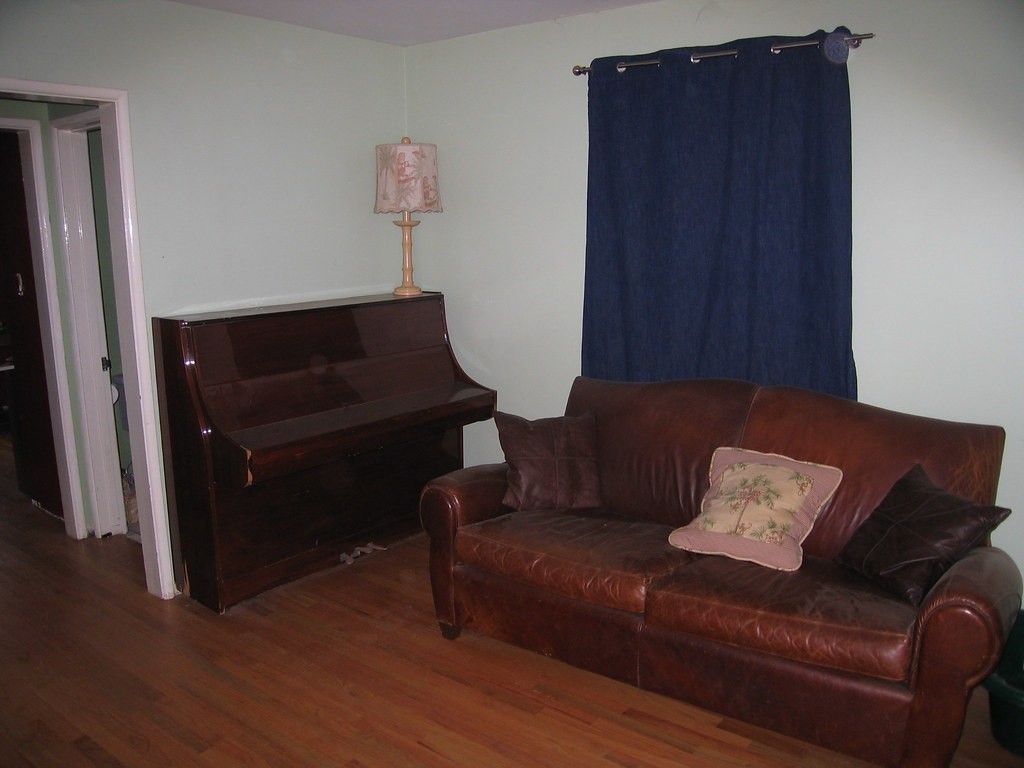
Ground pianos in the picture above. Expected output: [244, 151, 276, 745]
[150, 289, 498, 616]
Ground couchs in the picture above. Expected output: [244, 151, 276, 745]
[418, 376, 1024, 768]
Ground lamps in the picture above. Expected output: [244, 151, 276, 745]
[374, 135, 445, 297]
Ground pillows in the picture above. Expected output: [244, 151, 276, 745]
[492, 411, 603, 512]
[666, 447, 843, 572]
[831, 462, 1012, 608]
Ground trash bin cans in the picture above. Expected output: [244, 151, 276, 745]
[985, 607, 1024, 754]
